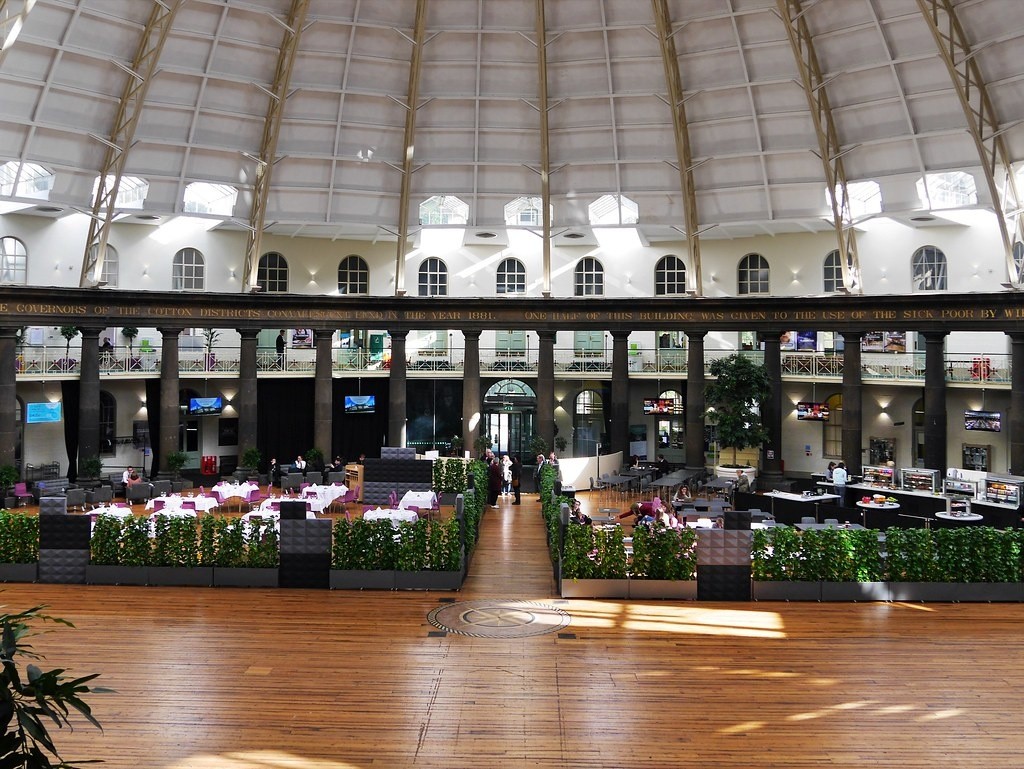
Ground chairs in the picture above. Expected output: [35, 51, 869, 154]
[14, 465, 443, 539]
[585, 465, 885, 539]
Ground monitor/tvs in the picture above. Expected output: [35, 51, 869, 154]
[189, 397, 223, 416]
[26, 402, 62, 424]
[344, 395, 377, 414]
[965, 410, 1001, 432]
[643, 398, 674, 416]
[797, 402, 830, 421]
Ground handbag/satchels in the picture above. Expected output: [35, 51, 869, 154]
[512, 480, 518, 487]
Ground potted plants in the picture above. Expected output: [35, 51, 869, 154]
[166, 449, 190, 493]
[239, 447, 261, 481]
[699, 353, 773, 484]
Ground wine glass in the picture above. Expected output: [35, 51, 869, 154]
[161, 492, 166, 498]
[99, 501, 105, 509]
[187, 492, 194, 499]
[62, 487, 65, 495]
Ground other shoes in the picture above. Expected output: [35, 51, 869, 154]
[490, 505, 500, 509]
[536, 499, 540, 502]
[512, 502, 520, 505]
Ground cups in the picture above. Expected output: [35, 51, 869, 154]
[802, 493, 808, 499]
[253, 505, 259, 512]
[223, 481, 227, 487]
[235, 480, 239, 486]
[270, 492, 276, 500]
[298, 493, 303, 499]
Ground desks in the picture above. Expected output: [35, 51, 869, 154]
[590, 516, 615, 525]
[663, 470, 702, 492]
[763, 492, 841, 522]
[145, 496, 219, 525]
[856, 500, 900, 528]
[85, 507, 132, 539]
[240, 509, 317, 540]
[676, 510, 724, 522]
[599, 476, 637, 502]
[302, 484, 348, 512]
[211, 483, 259, 512]
[621, 467, 656, 493]
[648, 478, 685, 503]
[672, 500, 732, 511]
[598, 507, 620, 517]
[793, 524, 868, 531]
[363, 507, 419, 543]
[398, 490, 436, 521]
[935, 511, 984, 529]
[147, 508, 197, 539]
[703, 476, 738, 499]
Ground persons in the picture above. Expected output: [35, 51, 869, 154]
[780, 332, 793, 347]
[480, 449, 502, 508]
[100, 338, 112, 363]
[626, 454, 638, 471]
[277, 330, 288, 367]
[673, 485, 691, 501]
[833, 462, 847, 507]
[294, 456, 306, 476]
[534, 452, 562, 502]
[731, 470, 749, 492]
[615, 497, 678, 535]
[356, 455, 366, 465]
[660, 333, 675, 348]
[508, 455, 523, 505]
[653, 455, 669, 480]
[826, 462, 836, 482]
[121, 466, 141, 486]
[501, 455, 514, 496]
[330, 458, 343, 472]
[712, 518, 725, 528]
[568, 498, 593, 530]
[269, 459, 288, 489]
[659, 438, 666, 448]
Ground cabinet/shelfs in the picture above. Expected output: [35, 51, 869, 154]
[345, 464, 364, 503]
[811, 466, 1024, 542]
[26, 461, 61, 480]
